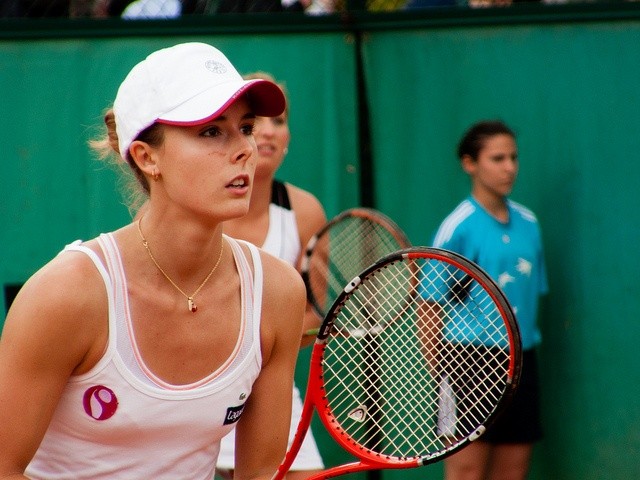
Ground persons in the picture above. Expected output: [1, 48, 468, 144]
[417, 120, 549, 480]
[0, 42, 307, 479]
[214, 71, 329, 479]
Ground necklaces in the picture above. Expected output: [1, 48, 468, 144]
[138, 217, 224, 313]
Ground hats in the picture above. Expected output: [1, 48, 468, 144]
[114, 43, 287, 190]
